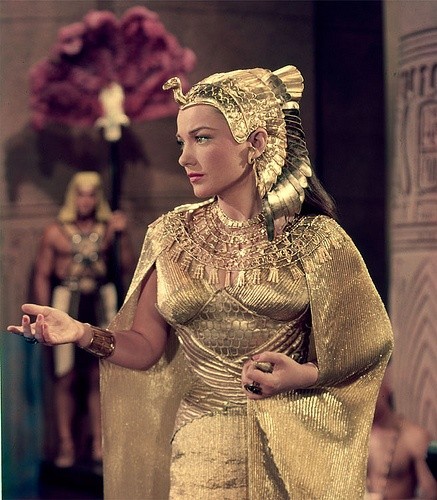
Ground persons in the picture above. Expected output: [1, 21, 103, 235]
[32, 172, 131, 469]
[366, 375, 436, 500]
[6, 65, 393, 500]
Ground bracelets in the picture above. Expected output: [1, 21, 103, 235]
[76, 323, 114, 359]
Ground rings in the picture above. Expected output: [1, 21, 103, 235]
[24, 337, 37, 345]
[243, 380, 262, 396]
